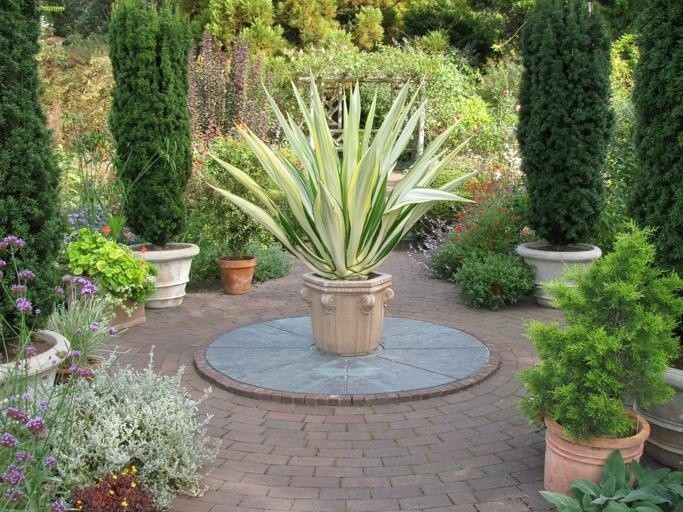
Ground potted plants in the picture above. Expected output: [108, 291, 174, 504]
[507, 13, 618, 310]
[0, 0, 69, 403]
[104, 0, 199, 309]
[199, 61, 481, 360]
[515, 216, 683, 502]
[190, 125, 304, 294]
